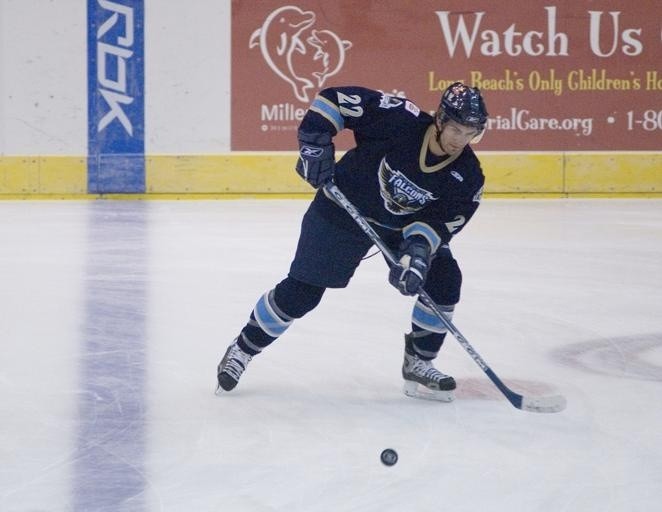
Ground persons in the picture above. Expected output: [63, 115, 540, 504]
[217, 81, 489, 391]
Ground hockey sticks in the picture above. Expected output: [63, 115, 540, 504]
[319, 180, 567, 414]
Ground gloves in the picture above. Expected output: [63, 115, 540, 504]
[295, 128, 335, 186]
[389, 235, 431, 296]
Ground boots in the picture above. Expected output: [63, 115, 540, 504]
[217, 343, 253, 391]
[402, 332, 456, 390]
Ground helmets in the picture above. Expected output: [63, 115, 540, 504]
[437, 82, 487, 135]
